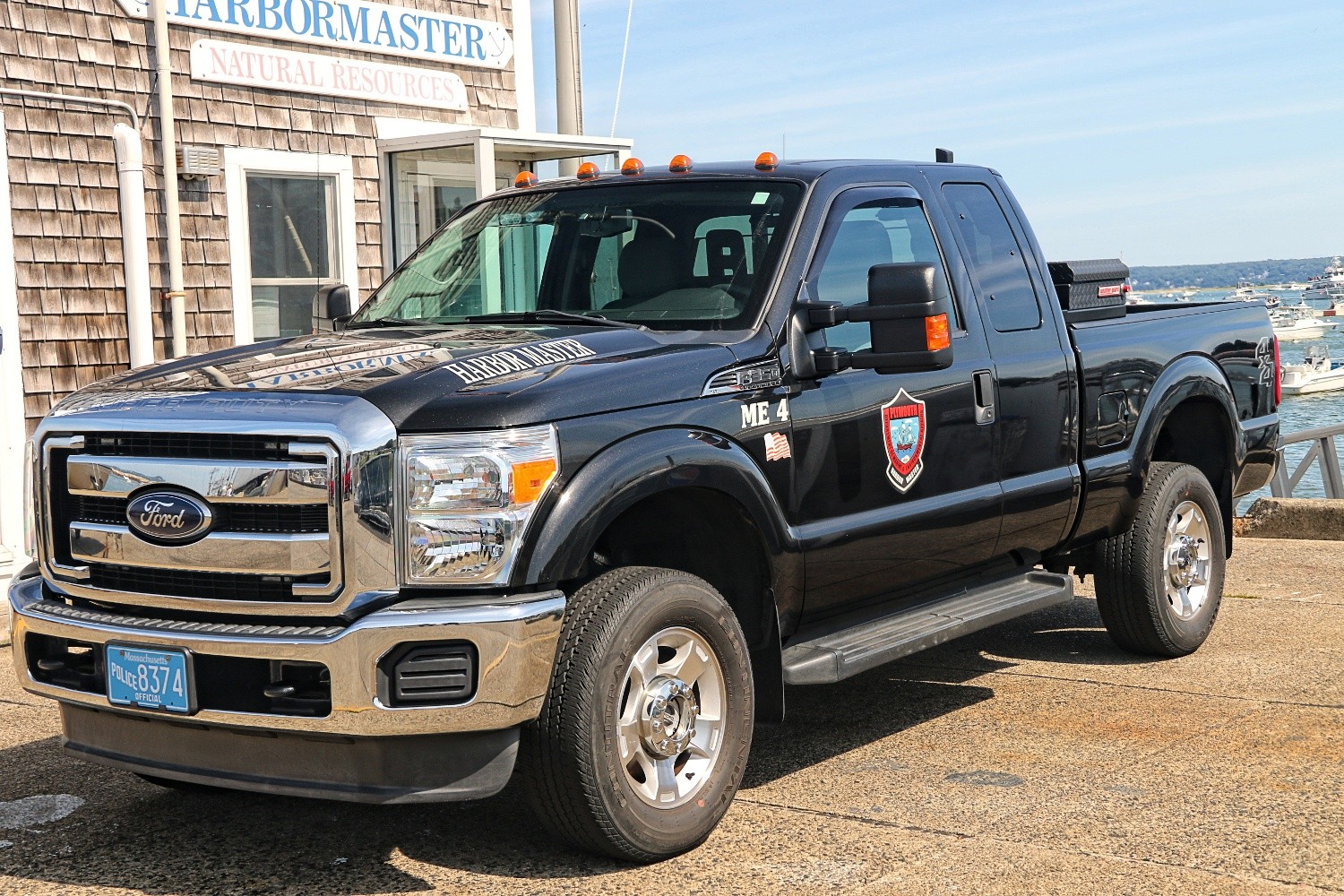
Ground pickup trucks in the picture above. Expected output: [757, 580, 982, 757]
[26, 150, 1295, 869]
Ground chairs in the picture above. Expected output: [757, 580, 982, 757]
[808, 220, 890, 349]
[601, 236, 679, 308]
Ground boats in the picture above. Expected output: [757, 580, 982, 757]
[1276, 339, 1344, 404]
[1266, 257, 1344, 343]
